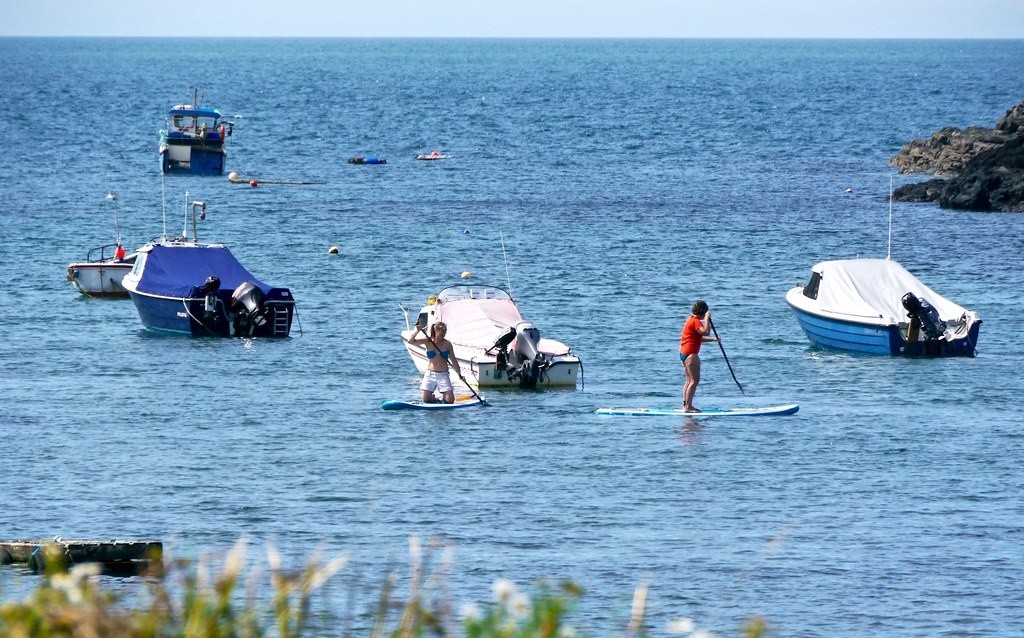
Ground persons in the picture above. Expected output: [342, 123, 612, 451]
[407, 321, 466, 404]
[679, 301, 721, 413]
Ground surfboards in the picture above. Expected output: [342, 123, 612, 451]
[596, 404, 800, 420]
[381, 395, 487, 411]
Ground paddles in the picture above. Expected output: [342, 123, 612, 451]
[414, 322, 490, 407]
[708, 317, 750, 397]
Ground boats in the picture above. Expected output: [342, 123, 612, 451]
[120, 164, 303, 341]
[400, 282, 580, 389]
[67, 192, 140, 299]
[159, 84, 235, 176]
[784, 174, 984, 359]
